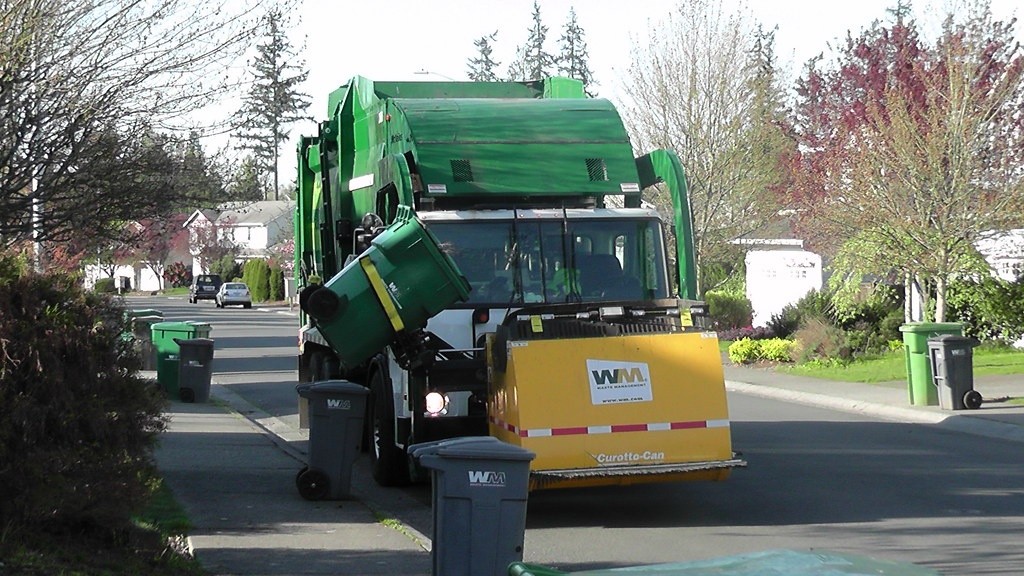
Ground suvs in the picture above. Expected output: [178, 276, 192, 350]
[189, 274, 224, 304]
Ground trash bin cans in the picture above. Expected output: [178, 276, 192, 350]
[295, 379, 373, 502]
[119, 276, 131, 290]
[172, 338, 216, 404]
[299, 204, 473, 372]
[407, 435, 538, 576]
[899, 321, 964, 407]
[151, 319, 213, 396]
[122, 308, 163, 371]
[926, 334, 982, 411]
[507, 548, 942, 576]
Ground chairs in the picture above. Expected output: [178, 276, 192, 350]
[582, 254, 623, 289]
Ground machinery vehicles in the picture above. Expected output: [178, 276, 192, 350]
[285, 74, 738, 485]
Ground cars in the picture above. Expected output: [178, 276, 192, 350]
[214, 282, 252, 309]
